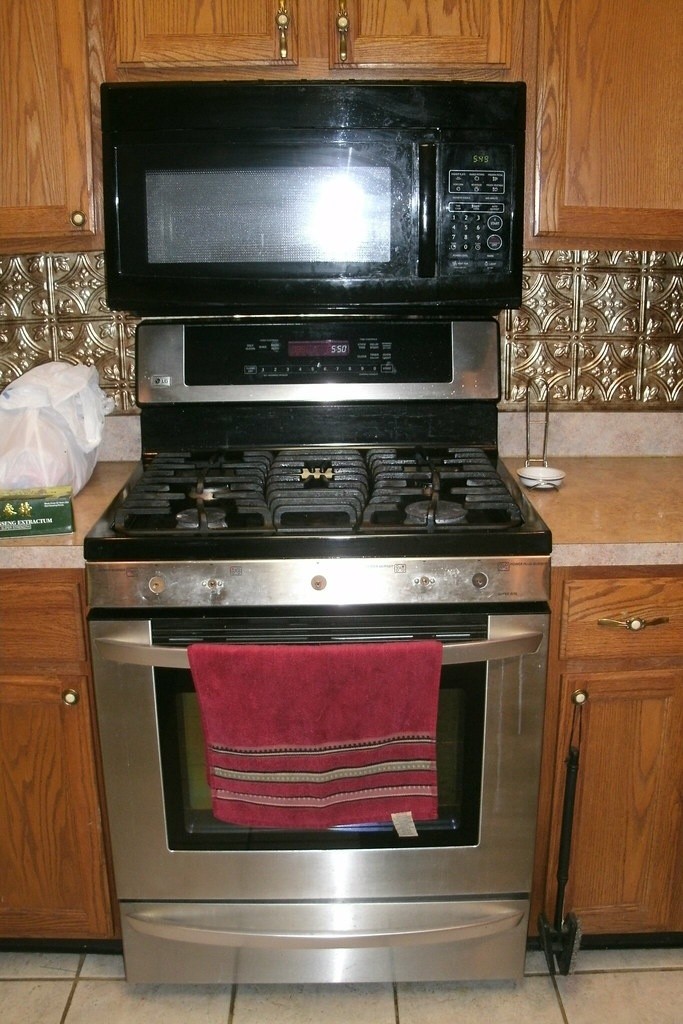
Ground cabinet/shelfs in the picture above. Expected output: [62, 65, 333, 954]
[0, 569, 118, 941]
[528, 564, 682, 939]
[0, 0, 105, 255]
[523, 0, 683, 252]
[100, 0, 523, 81]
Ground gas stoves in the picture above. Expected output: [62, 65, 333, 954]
[84, 315, 555, 559]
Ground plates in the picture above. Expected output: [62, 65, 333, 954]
[517, 466, 565, 488]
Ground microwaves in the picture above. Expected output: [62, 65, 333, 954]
[99, 81, 527, 313]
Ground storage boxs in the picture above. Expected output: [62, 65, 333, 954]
[0, 485, 76, 537]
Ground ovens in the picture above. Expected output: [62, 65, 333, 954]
[84, 563, 552, 986]
